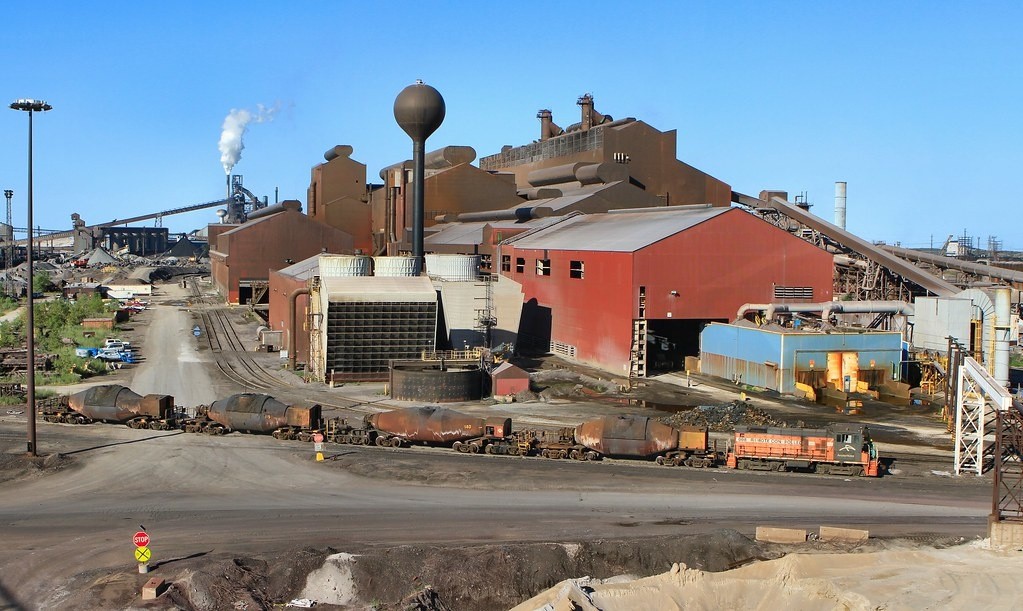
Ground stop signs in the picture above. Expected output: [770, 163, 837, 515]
[314, 434, 323, 443]
[133, 532, 150, 547]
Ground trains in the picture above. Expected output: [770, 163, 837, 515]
[43, 383, 883, 477]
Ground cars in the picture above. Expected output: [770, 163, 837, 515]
[76, 338, 136, 364]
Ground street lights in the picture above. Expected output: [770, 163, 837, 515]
[11, 98, 53, 459]
